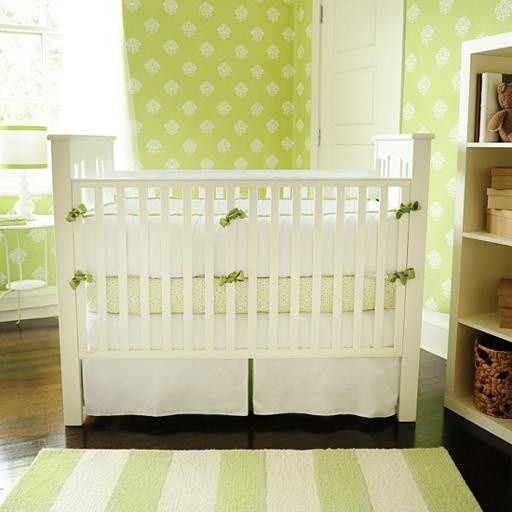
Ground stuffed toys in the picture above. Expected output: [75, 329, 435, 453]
[486, 81, 512, 142]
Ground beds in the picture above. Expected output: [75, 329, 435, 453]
[47, 133, 436, 431]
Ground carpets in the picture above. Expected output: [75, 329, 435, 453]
[0, 443, 486, 512]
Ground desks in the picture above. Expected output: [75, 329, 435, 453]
[1, 216, 53, 332]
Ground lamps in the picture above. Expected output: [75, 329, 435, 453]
[0, 124, 48, 219]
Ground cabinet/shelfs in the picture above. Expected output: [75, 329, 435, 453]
[443, 33, 512, 451]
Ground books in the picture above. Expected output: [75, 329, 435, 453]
[479, 71, 512, 144]
[473, 72, 482, 144]
